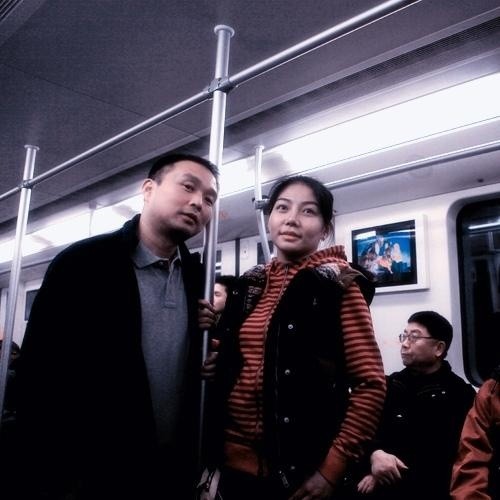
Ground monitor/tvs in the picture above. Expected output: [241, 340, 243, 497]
[347, 214, 431, 293]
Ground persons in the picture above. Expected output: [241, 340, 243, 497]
[350, 310, 476, 500]
[195, 175, 389, 498]
[1, 328, 19, 375]
[210, 276, 237, 381]
[450, 377, 499, 500]
[363, 236, 407, 274]
[0, 153, 231, 500]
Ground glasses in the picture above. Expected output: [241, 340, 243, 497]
[398, 332, 435, 344]
